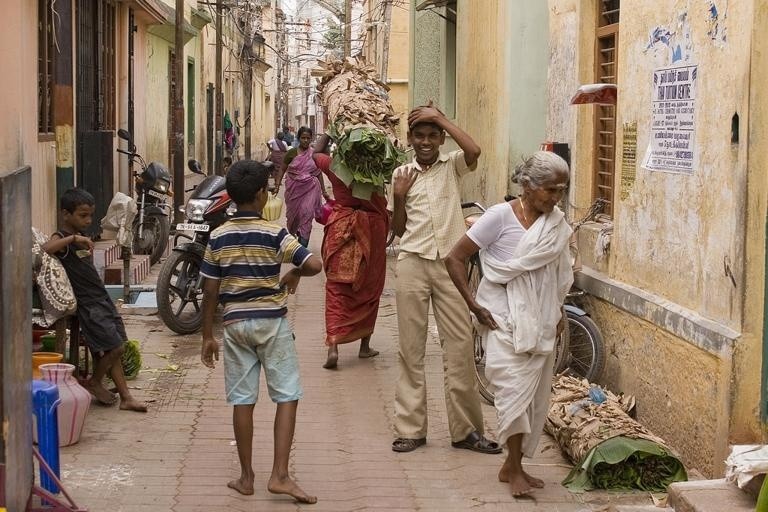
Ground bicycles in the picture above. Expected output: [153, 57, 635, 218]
[385, 227, 396, 258]
[453, 194, 569, 407]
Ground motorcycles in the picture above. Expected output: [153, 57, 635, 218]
[155, 157, 276, 337]
[113, 128, 174, 267]
[554, 288, 609, 386]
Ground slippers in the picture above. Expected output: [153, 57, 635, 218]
[392, 438, 426, 452]
[451, 432, 503, 454]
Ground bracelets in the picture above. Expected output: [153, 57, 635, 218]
[275, 186, 279, 189]
[72, 233, 76, 244]
[322, 191, 327, 193]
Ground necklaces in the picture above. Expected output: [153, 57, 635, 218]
[416, 157, 438, 169]
[519, 197, 531, 229]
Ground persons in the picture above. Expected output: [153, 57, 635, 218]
[312, 127, 391, 369]
[267, 126, 294, 185]
[391, 104, 505, 453]
[445, 151, 573, 498]
[223, 157, 232, 173]
[272, 126, 330, 247]
[195, 161, 320, 504]
[42, 186, 149, 412]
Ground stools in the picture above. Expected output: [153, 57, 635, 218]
[32, 380, 61, 505]
